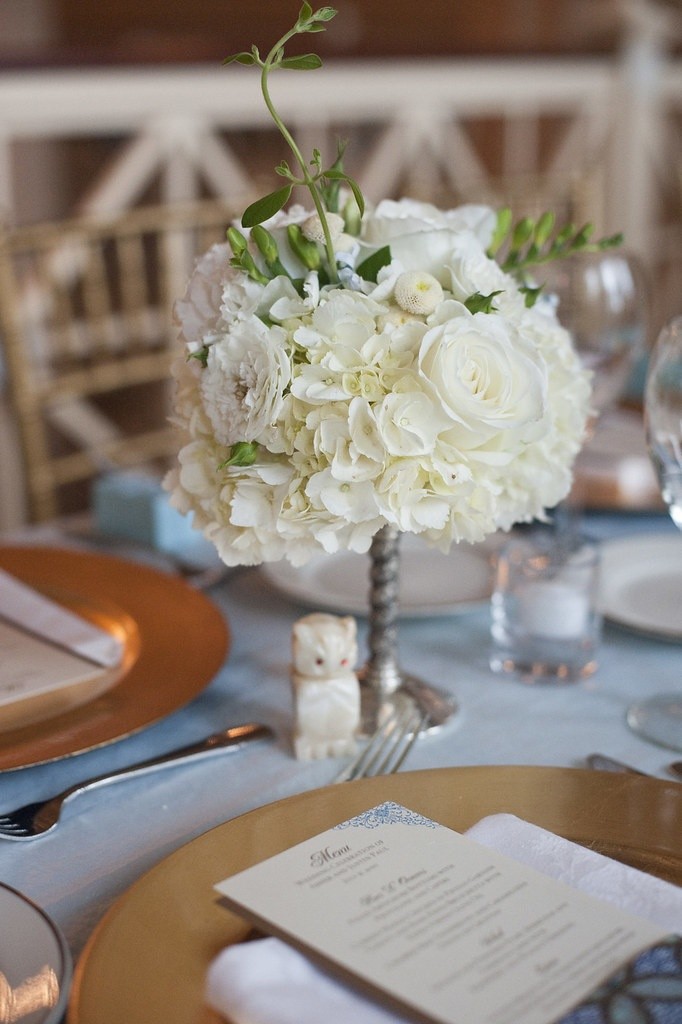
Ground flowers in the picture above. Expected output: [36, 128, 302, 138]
[158, 4, 625, 566]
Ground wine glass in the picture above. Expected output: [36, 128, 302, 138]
[626, 317, 682, 753]
[519, 250, 648, 567]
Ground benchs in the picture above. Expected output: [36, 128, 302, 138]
[2, 60, 681, 523]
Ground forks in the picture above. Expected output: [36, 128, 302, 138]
[0, 722, 274, 840]
[336, 705, 429, 785]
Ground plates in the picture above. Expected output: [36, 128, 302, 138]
[253, 531, 535, 618]
[1, 545, 228, 774]
[67, 765, 682, 1024]
[599, 533, 681, 644]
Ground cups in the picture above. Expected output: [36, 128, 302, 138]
[490, 531, 601, 685]
[0, 881, 72, 1024]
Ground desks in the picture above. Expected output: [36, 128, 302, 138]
[1, 506, 682, 1024]
